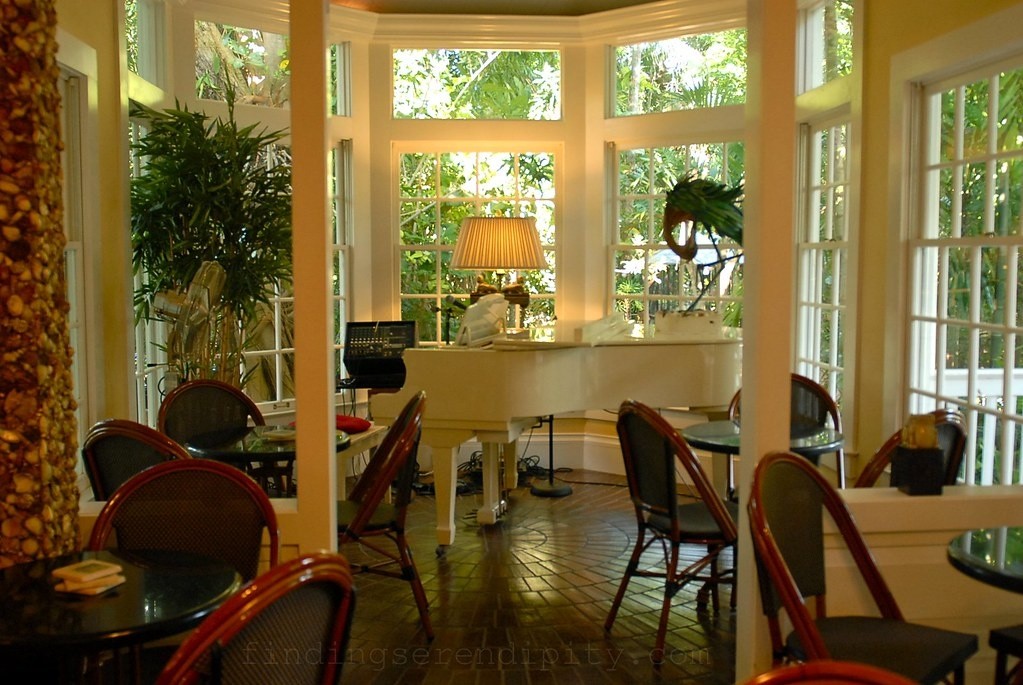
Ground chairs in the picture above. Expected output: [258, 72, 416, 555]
[602, 398, 740, 663]
[156, 551, 359, 685]
[157, 379, 286, 499]
[725, 373, 848, 506]
[336, 390, 436, 642]
[82, 419, 197, 503]
[79, 459, 280, 685]
[748, 448, 979, 684]
[853, 408, 968, 490]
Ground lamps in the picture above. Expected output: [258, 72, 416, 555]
[446, 216, 549, 337]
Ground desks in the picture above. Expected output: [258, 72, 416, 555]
[680, 416, 845, 613]
[945, 526, 1022, 685]
[0, 548, 242, 685]
[183, 423, 351, 500]
[252, 414, 391, 531]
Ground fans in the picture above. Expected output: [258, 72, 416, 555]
[152, 260, 228, 387]
